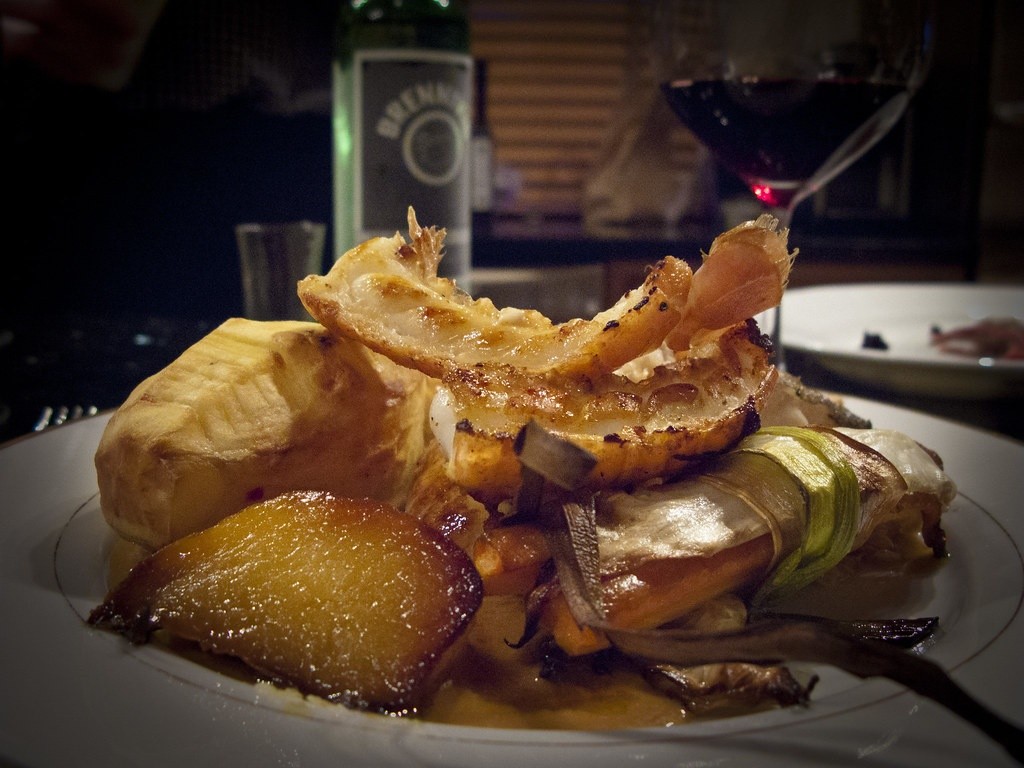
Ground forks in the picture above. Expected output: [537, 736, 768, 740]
[33, 405, 98, 433]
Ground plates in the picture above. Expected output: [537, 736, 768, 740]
[752, 282, 1024, 404]
[2, 390, 1024, 767]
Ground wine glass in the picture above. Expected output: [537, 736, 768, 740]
[658, 0, 922, 375]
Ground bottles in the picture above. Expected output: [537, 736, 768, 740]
[331, 1, 477, 295]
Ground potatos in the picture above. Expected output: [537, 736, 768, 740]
[86, 492, 485, 716]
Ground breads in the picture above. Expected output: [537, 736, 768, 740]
[96, 316, 431, 551]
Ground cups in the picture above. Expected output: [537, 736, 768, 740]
[236, 223, 326, 322]
